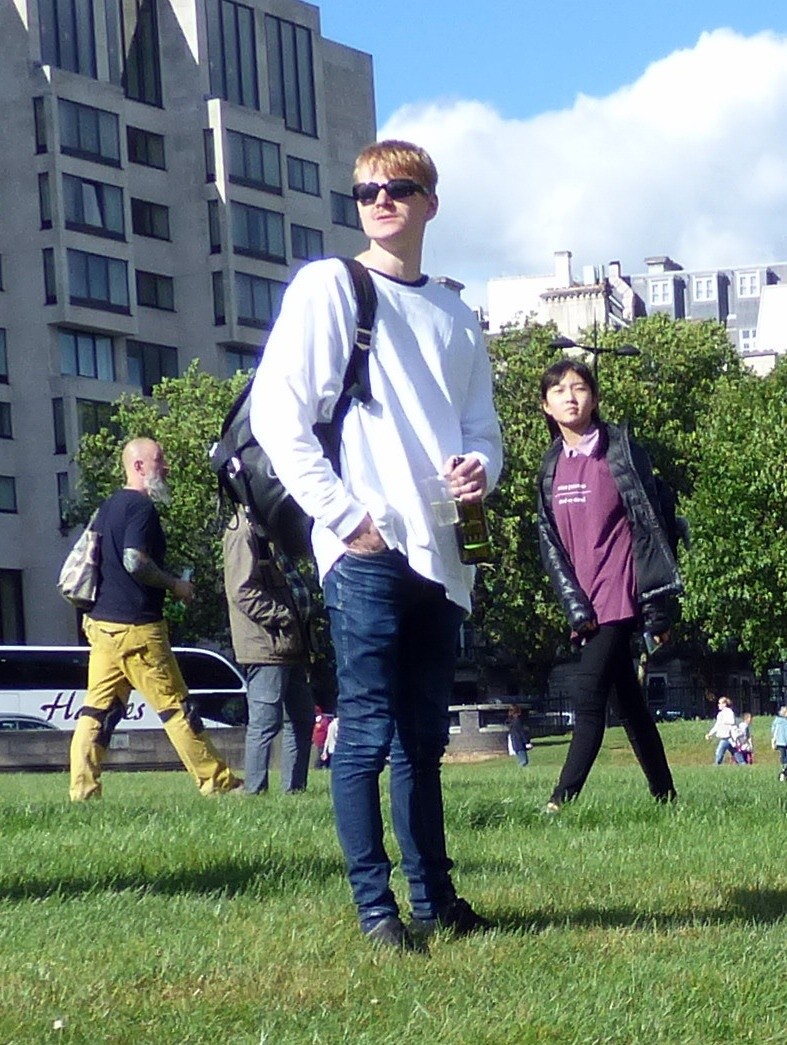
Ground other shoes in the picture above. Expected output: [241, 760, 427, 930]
[408, 897, 500, 941]
[363, 914, 429, 959]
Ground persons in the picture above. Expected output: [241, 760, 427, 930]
[771, 706, 787, 783]
[705, 696, 753, 766]
[247, 142, 505, 963]
[68, 437, 246, 801]
[533, 359, 682, 815]
[311, 703, 339, 767]
[223, 500, 316, 796]
[507, 704, 532, 767]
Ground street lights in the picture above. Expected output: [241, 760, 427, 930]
[548, 335, 643, 394]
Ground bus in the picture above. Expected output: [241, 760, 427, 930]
[1, 644, 253, 737]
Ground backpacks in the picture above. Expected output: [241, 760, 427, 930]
[210, 255, 378, 556]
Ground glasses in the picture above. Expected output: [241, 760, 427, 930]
[352, 179, 431, 203]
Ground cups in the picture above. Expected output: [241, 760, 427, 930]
[419, 474, 460, 526]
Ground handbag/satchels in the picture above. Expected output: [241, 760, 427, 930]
[57, 506, 100, 611]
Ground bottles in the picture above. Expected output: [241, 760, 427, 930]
[180, 566, 191, 583]
[452, 456, 493, 564]
